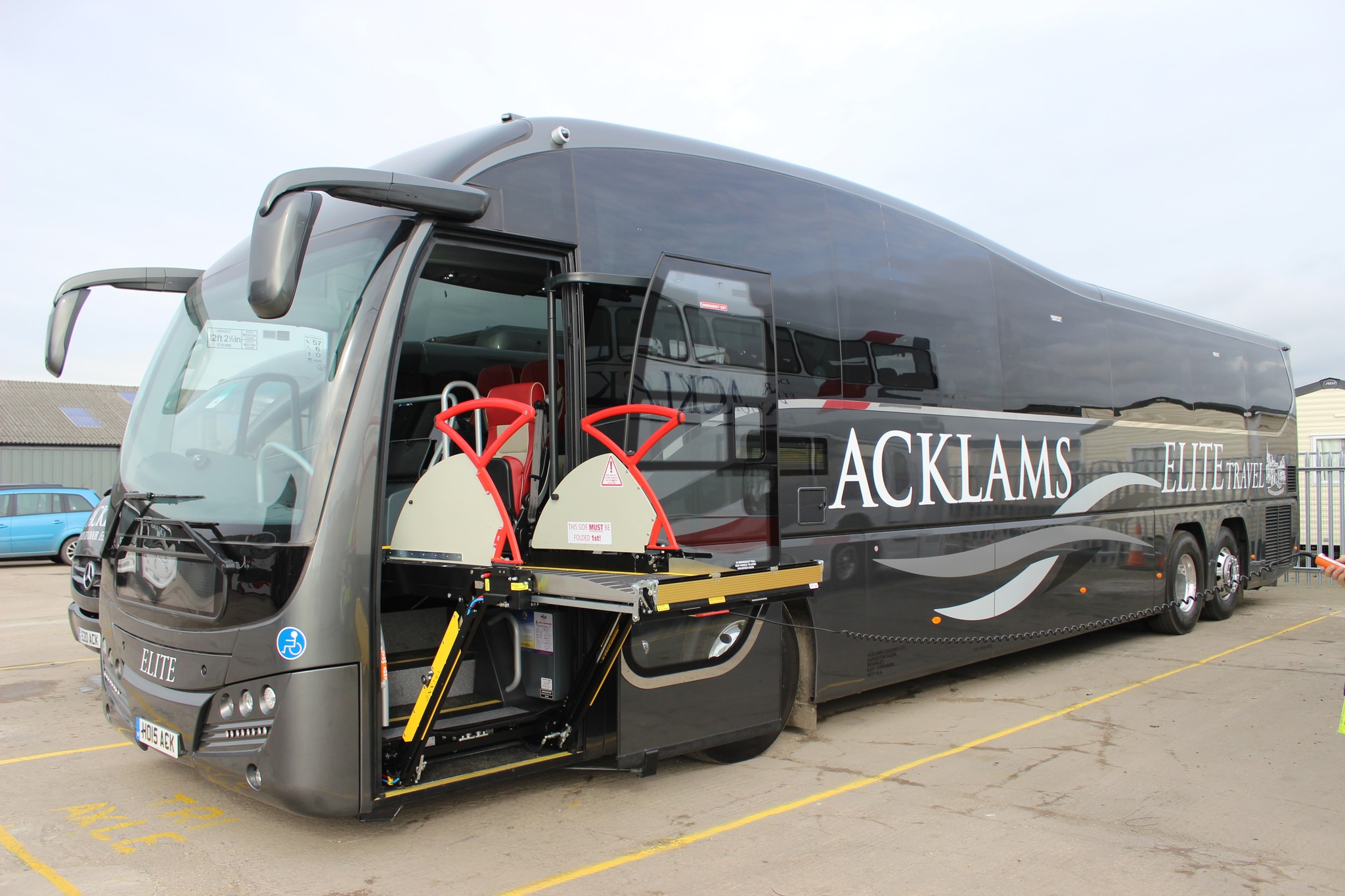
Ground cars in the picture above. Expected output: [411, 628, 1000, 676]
[1, 486, 103, 567]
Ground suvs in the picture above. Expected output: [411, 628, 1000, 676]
[68, 486, 113, 653]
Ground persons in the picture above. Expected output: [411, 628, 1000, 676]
[1320, 554, 1345, 735]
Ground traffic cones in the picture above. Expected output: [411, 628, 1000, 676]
[1119, 524, 1145, 570]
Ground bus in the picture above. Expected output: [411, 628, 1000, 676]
[42, 111, 1300, 819]
